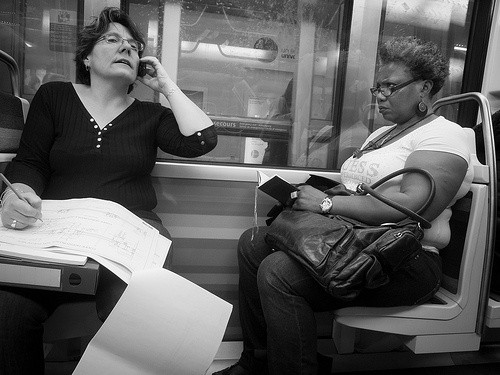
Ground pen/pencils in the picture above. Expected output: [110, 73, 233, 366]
[0, 173, 45, 223]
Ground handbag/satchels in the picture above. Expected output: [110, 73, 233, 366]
[264, 167, 435, 302]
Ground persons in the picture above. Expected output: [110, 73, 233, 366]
[214, 35, 474, 375]
[0, 6, 218, 375]
[261, 50, 372, 171]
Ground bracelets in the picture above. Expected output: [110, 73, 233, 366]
[0, 188, 24, 208]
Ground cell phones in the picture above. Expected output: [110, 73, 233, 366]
[138, 61, 147, 76]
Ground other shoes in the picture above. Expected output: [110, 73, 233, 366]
[212, 362, 250, 375]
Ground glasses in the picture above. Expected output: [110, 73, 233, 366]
[371, 75, 429, 97]
[93, 34, 144, 51]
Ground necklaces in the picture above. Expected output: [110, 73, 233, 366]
[353, 112, 435, 157]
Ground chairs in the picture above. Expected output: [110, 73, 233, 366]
[335, 92, 500, 354]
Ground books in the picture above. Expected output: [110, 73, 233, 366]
[256, 171, 301, 206]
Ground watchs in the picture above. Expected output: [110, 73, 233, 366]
[320, 194, 334, 216]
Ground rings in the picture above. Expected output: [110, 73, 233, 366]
[11, 219, 17, 229]
[290, 191, 298, 200]
